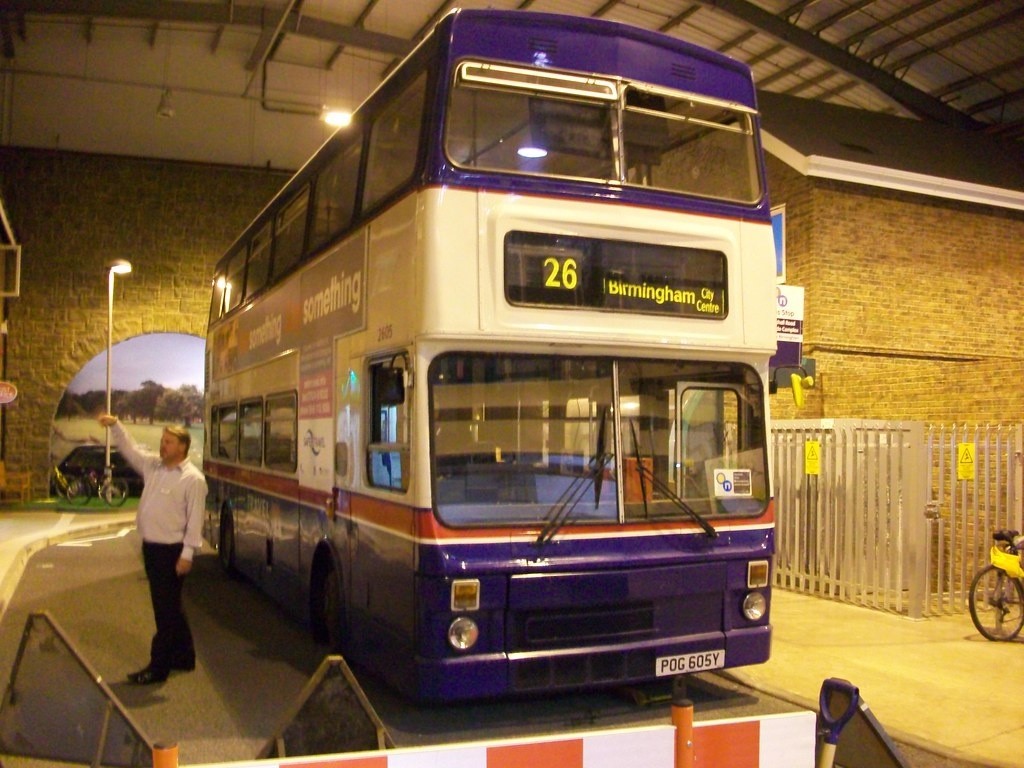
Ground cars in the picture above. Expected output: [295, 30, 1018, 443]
[58, 444, 148, 494]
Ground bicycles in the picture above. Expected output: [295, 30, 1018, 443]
[967, 529, 1024, 643]
[65, 465, 129, 508]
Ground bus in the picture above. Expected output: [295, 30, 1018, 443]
[200, 11, 816, 712]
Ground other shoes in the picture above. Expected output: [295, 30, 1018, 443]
[128, 663, 168, 685]
[170, 655, 195, 670]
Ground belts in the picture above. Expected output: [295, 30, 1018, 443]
[142, 543, 182, 549]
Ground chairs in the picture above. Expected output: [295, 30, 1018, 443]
[375, 442, 619, 511]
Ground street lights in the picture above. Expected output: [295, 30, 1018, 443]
[104, 258, 132, 483]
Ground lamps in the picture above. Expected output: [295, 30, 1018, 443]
[156, 85, 176, 121]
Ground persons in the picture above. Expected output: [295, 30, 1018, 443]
[100, 415, 208, 683]
